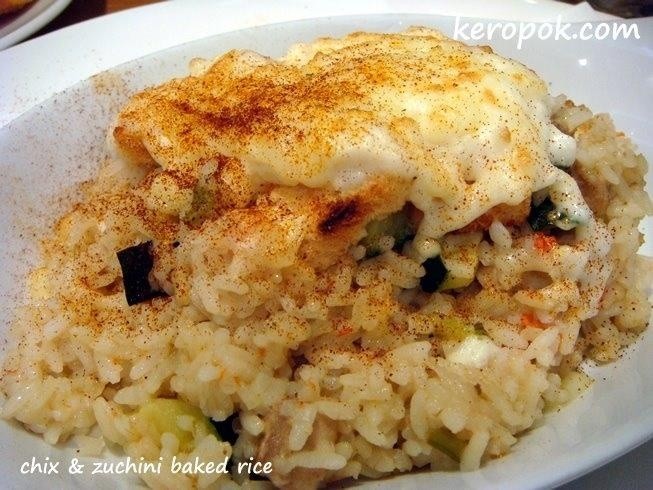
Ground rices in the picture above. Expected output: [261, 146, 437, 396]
[0, 93, 653, 490]
